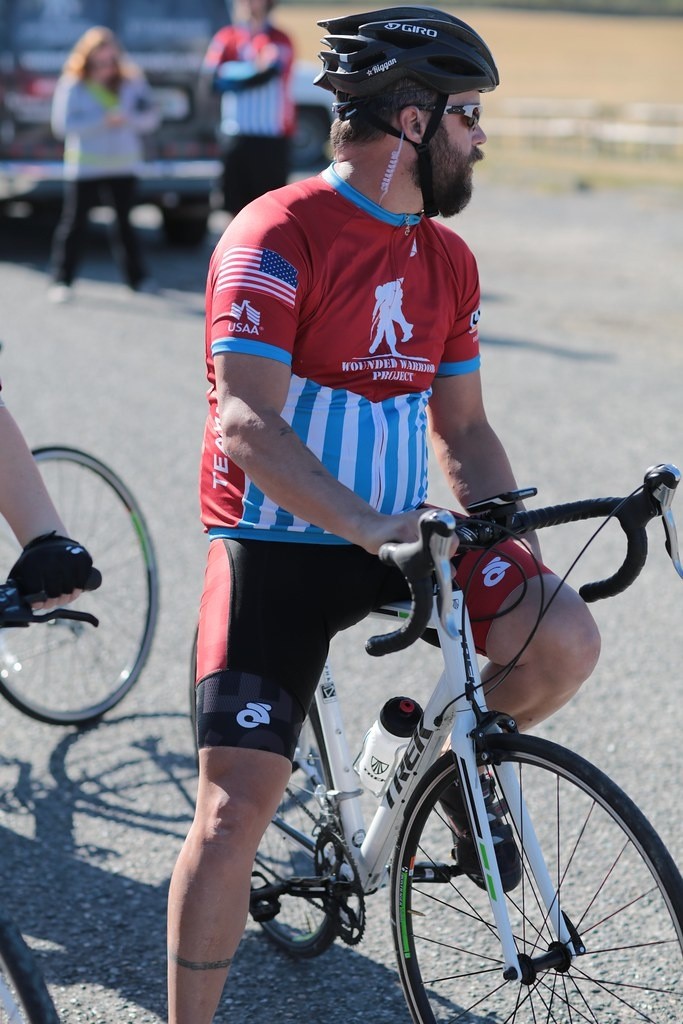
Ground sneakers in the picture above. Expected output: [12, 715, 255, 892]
[437, 772, 522, 892]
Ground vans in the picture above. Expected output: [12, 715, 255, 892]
[1, 0, 225, 260]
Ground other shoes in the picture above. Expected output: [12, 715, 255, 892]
[51, 287, 71, 301]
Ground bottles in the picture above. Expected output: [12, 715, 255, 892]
[359, 696, 423, 796]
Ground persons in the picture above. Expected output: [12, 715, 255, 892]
[47, 26, 163, 304]
[0, 399, 85, 612]
[167, 7, 602, 1024]
[196, 0, 297, 219]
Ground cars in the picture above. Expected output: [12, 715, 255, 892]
[286, 65, 339, 171]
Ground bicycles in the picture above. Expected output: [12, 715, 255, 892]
[190, 461, 683, 1024]
[0, 447, 159, 724]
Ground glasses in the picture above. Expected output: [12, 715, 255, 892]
[398, 103, 483, 131]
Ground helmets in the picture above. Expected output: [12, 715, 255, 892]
[313, 5, 500, 121]
